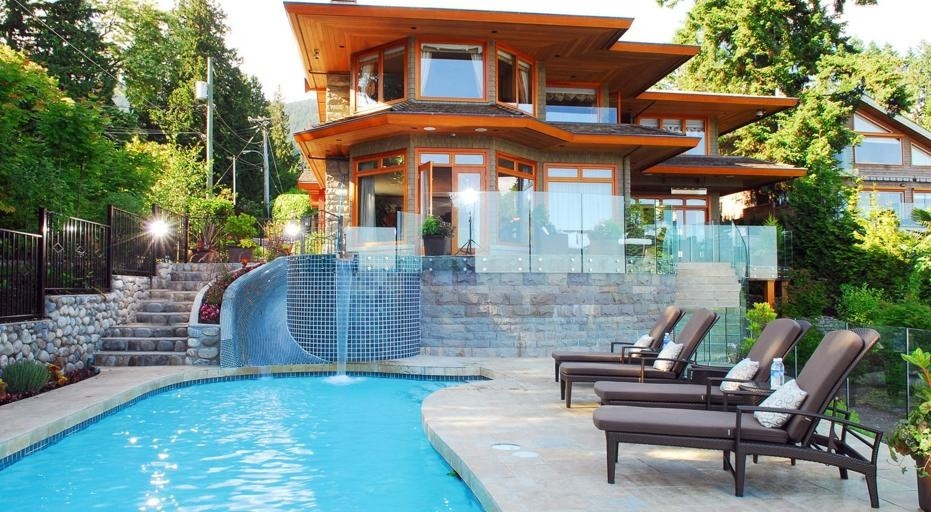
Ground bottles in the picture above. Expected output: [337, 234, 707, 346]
[663, 333, 671, 348]
[770, 357, 784, 390]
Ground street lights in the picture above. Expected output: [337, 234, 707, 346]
[452, 188, 480, 255]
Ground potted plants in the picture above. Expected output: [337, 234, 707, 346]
[186, 221, 257, 263]
[422, 216, 457, 256]
[886, 348, 931, 512]
